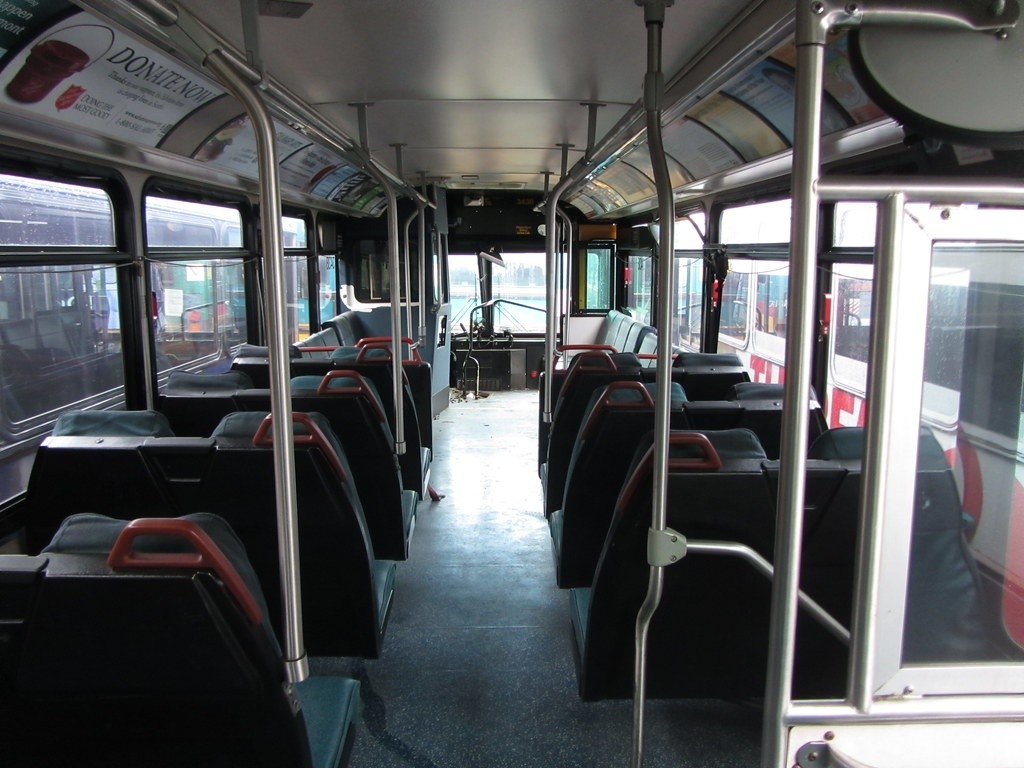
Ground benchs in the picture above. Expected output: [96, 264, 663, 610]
[539, 310, 989, 704]
[0, 312, 432, 768]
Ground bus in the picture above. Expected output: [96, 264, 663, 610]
[0, 178, 314, 501]
[222, 215, 340, 343]
[628, 196, 1024, 606]
[169, 256, 231, 334]
